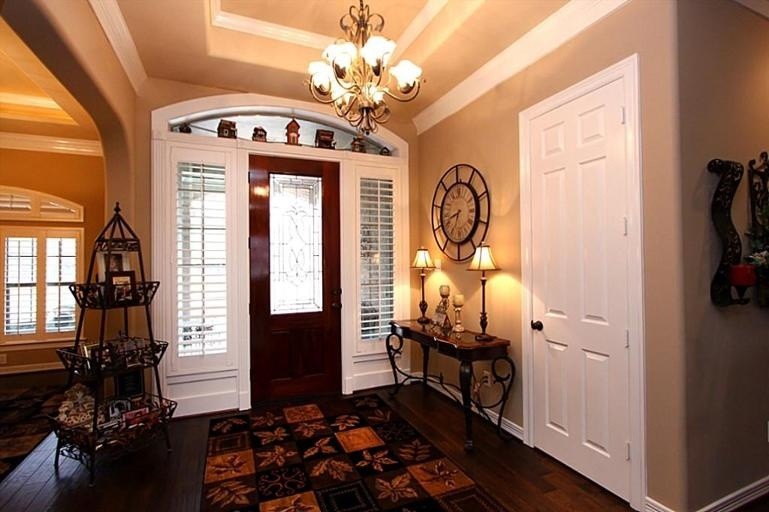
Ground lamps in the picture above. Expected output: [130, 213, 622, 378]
[465, 242, 501, 342]
[409, 246, 436, 324]
[303, 0, 423, 138]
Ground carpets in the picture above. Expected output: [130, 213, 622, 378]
[200, 393, 509, 512]
[0, 385, 68, 486]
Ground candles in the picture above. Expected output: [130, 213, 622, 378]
[453, 294, 465, 306]
[440, 285, 450, 297]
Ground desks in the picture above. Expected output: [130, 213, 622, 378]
[385, 320, 515, 453]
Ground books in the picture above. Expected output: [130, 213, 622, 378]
[73, 334, 160, 365]
[91, 396, 157, 444]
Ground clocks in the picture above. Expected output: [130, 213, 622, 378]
[431, 164, 490, 262]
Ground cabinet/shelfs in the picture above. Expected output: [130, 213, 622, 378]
[45, 199, 177, 488]
